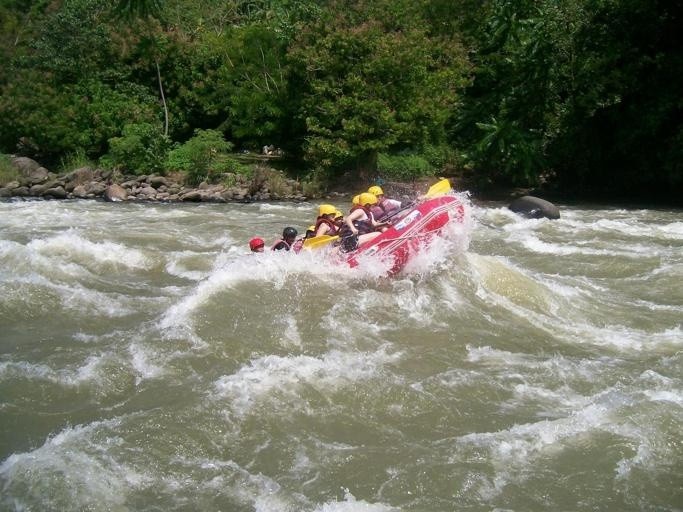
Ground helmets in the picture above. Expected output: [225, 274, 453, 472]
[367, 185, 383, 195]
[282, 227, 297, 238]
[334, 210, 342, 219]
[319, 204, 336, 217]
[249, 238, 264, 249]
[358, 192, 377, 206]
[352, 194, 358, 204]
[306, 225, 315, 232]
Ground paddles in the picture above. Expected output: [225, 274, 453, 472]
[304, 223, 388, 248]
[379, 179, 451, 222]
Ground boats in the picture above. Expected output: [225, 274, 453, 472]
[325, 194, 465, 280]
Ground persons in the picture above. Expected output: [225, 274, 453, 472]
[249, 185, 425, 262]
